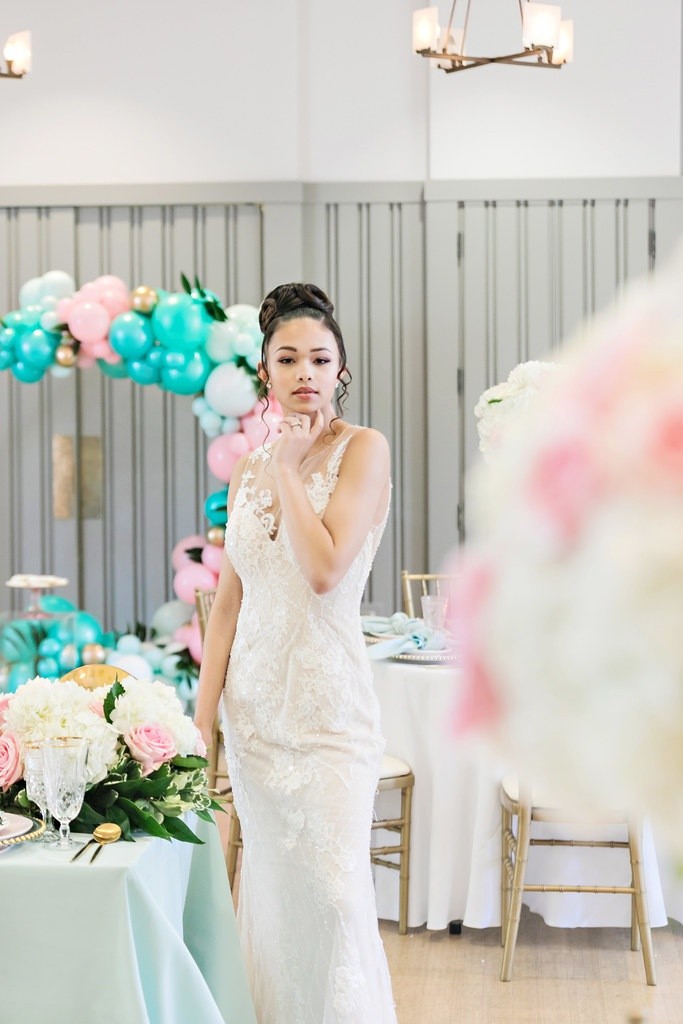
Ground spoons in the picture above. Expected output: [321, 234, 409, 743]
[90, 823, 121, 865]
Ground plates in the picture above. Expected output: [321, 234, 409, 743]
[0, 816, 45, 847]
[0, 811, 33, 841]
[393, 651, 465, 666]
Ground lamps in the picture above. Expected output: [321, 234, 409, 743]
[414, 1, 574, 75]
[0, 30, 33, 79]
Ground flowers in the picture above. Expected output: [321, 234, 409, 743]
[0, 677, 230, 863]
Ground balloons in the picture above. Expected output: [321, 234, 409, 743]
[0, 270, 283, 712]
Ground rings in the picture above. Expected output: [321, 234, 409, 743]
[291, 422, 301, 427]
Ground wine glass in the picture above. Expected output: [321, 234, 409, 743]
[26, 744, 61, 844]
[44, 737, 90, 850]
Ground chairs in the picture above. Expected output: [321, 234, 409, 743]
[196, 587, 416, 932]
[399, 570, 448, 621]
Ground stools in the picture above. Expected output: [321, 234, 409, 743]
[494, 777, 657, 988]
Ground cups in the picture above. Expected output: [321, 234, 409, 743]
[420, 595, 448, 629]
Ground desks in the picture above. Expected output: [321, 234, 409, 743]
[368, 654, 682, 930]
[0, 806, 238, 1022]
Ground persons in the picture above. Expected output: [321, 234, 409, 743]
[195, 282, 396, 1024]
